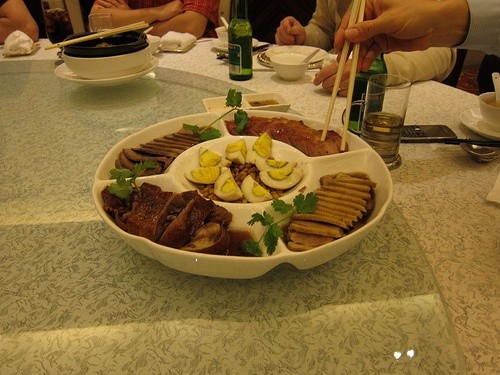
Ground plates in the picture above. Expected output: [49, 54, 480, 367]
[264, 46, 326, 64]
[55, 55, 159, 86]
[92, 109, 394, 277]
[459, 107, 500, 142]
[210, 38, 259, 52]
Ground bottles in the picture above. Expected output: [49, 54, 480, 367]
[227, 0, 254, 81]
[348, 52, 387, 136]
[41, 0, 74, 43]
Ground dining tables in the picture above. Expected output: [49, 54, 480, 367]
[0, 38, 500, 375]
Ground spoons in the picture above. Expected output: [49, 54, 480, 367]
[459, 142, 500, 159]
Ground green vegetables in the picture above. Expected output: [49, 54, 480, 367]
[183, 88, 248, 140]
[106, 160, 160, 201]
[240, 190, 319, 256]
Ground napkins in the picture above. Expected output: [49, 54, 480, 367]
[162, 31, 197, 49]
[4, 30, 34, 51]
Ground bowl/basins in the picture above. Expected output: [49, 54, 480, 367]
[147, 36, 161, 54]
[269, 53, 310, 81]
[477, 92, 500, 132]
[215, 26, 229, 43]
[62, 31, 147, 57]
[62, 41, 151, 79]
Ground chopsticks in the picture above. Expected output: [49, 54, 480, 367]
[252, 68, 275, 72]
[45, 21, 150, 51]
[320, 0, 366, 151]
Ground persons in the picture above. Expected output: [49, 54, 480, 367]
[275, 0, 457, 97]
[0, 0, 39, 43]
[335, 0, 500, 74]
[90, 0, 219, 39]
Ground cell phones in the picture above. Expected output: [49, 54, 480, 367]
[399, 124, 457, 143]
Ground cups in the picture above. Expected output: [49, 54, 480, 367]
[201, 96, 251, 113]
[359, 74, 412, 171]
[242, 91, 291, 113]
[88, 12, 113, 31]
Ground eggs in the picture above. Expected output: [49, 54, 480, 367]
[241, 175, 272, 203]
[258, 170, 303, 190]
[245, 131, 272, 164]
[213, 168, 243, 201]
[183, 167, 229, 184]
[199, 146, 232, 167]
[254, 158, 302, 172]
